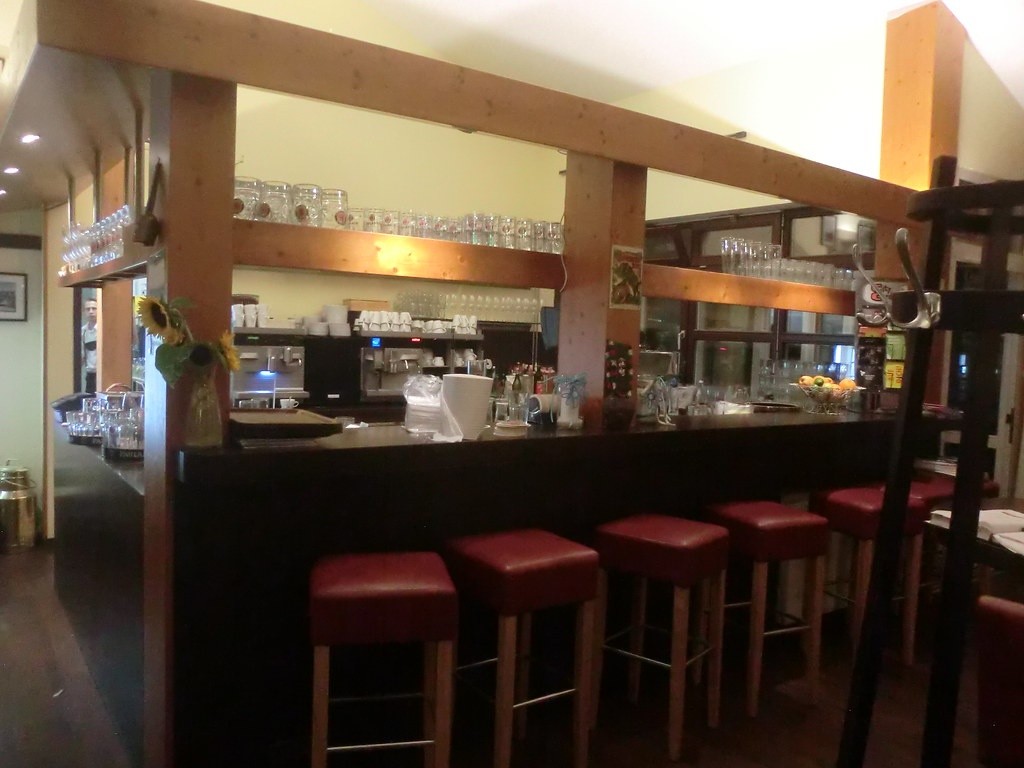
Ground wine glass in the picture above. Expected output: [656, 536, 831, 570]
[446, 294, 543, 323]
[60, 217, 91, 272]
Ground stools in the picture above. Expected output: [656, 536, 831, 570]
[445, 528, 600, 768]
[696, 501, 829, 717]
[599, 516, 729, 763]
[308, 551, 457, 768]
[824, 470, 955, 667]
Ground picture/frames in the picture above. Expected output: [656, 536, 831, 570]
[0, 272, 28, 321]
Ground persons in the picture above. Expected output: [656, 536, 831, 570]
[80, 298, 97, 398]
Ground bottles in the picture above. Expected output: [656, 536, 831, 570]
[738, 387, 750, 404]
[512, 373, 522, 402]
[693, 380, 707, 415]
[87, 203, 132, 265]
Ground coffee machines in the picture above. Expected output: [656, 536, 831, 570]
[359, 347, 423, 398]
[231, 344, 310, 399]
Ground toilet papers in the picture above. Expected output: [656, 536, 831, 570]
[529, 395, 558, 413]
[430, 373, 493, 443]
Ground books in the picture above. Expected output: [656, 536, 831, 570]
[926, 508, 1024, 555]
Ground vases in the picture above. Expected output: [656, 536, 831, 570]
[185, 362, 223, 446]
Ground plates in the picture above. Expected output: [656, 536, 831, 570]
[308, 321, 327, 336]
[405, 395, 441, 432]
[442, 373, 493, 440]
[326, 305, 351, 337]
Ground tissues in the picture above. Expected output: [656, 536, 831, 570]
[402, 374, 442, 432]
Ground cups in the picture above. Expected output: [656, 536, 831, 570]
[781, 256, 856, 292]
[353, 310, 478, 335]
[456, 347, 478, 366]
[65, 397, 104, 435]
[432, 356, 444, 366]
[759, 359, 849, 405]
[99, 408, 146, 449]
[230, 303, 271, 328]
[507, 391, 530, 424]
[530, 394, 559, 413]
[231, 175, 565, 254]
[494, 399, 508, 421]
[279, 399, 300, 409]
[334, 415, 355, 426]
[720, 236, 781, 280]
